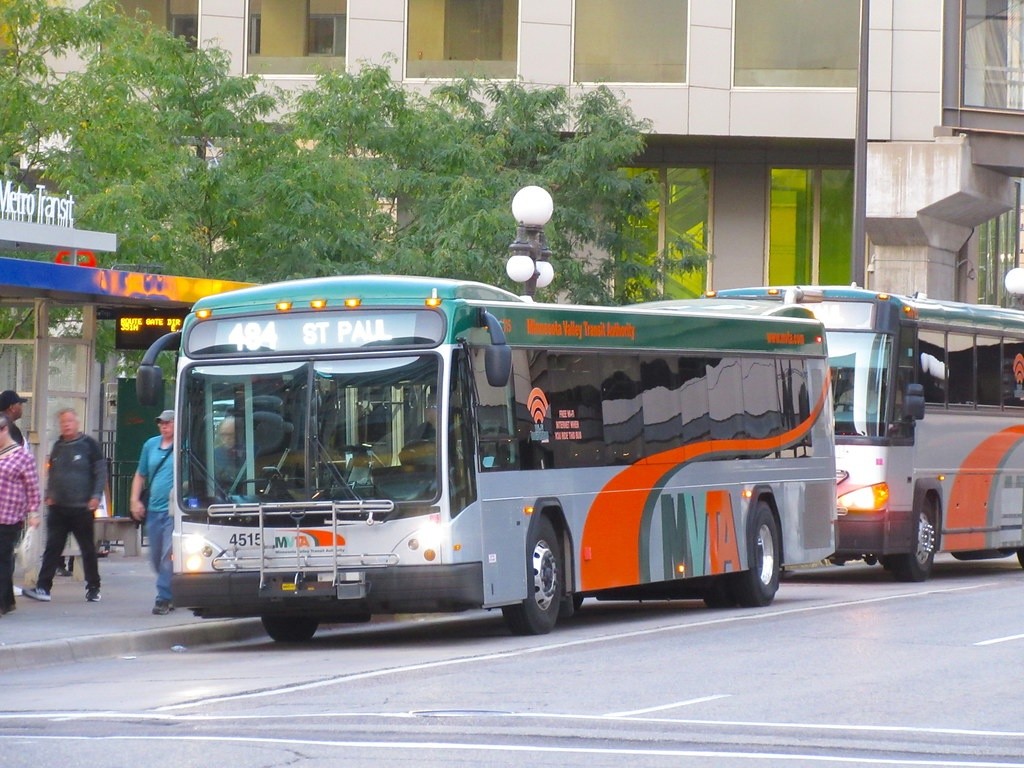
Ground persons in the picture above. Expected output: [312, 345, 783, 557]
[129, 410, 190, 615]
[213, 416, 274, 496]
[396, 392, 460, 481]
[834, 368, 879, 415]
[21, 408, 106, 602]
[0, 416, 40, 617]
[0, 390, 29, 597]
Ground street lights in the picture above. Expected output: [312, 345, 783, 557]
[506, 184, 554, 303]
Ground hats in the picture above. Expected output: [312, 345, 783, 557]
[154, 410, 175, 422]
[0, 390, 28, 410]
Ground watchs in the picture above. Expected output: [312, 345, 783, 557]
[29, 513, 40, 518]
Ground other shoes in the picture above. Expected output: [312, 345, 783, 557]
[0, 602, 17, 617]
[152, 600, 169, 614]
[167, 600, 174, 611]
[55, 568, 71, 576]
[12, 585, 23, 595]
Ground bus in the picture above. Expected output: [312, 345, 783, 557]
[136, 275, 839, 642]
[701, 284, 1023, 585]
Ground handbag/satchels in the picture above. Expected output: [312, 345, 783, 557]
[130, 487, 150, 527]
[12, 524, 39, 590]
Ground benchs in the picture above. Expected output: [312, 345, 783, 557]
[94, 517, 141, 556]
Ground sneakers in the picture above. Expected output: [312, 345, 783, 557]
[23, 587, 51, 600]
[85, 588, 101, 601]
